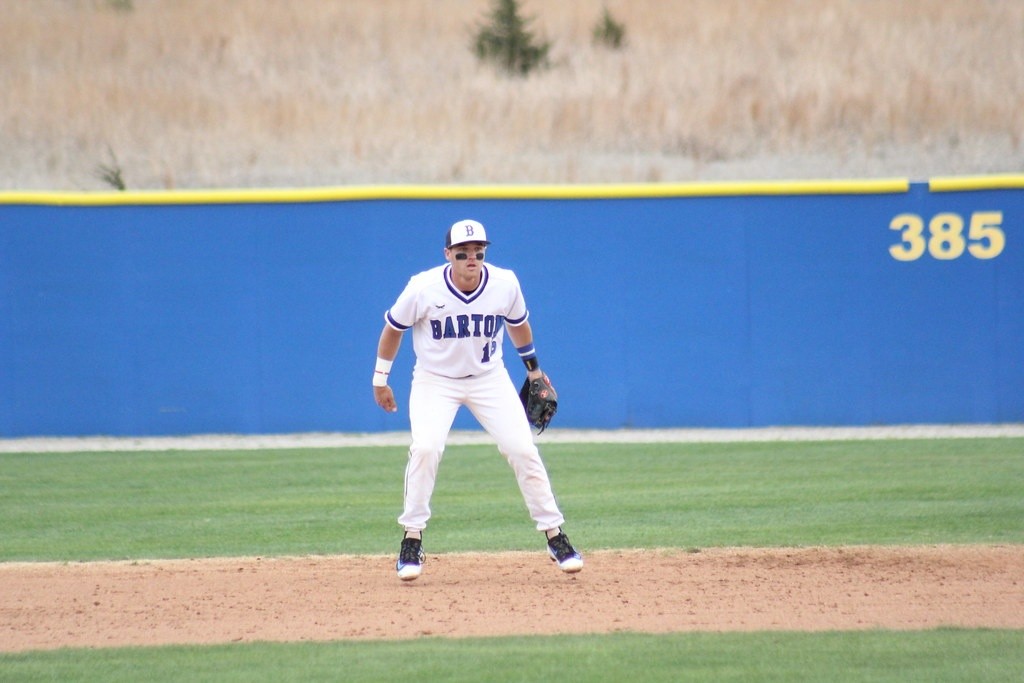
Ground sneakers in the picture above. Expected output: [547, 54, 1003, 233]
[396, 531, 424, 580]
[545, 526, 584, 574]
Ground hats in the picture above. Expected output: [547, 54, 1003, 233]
[446, 219, 491, 248]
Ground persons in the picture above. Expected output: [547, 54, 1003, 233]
[373, 221, 583, 581]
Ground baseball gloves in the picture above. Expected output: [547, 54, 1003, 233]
[518, 374, 558, 429]
